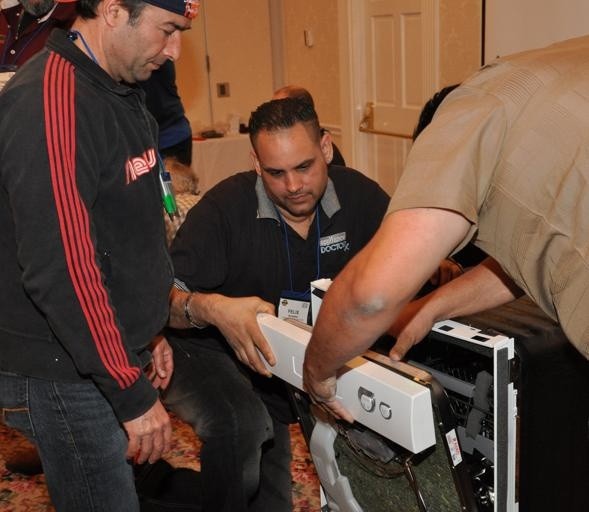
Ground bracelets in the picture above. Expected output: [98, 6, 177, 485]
[180, 289, 207, 332]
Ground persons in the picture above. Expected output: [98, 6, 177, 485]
[1, 0, 87, 92]
[268, 82, 349, 169]
[301, 32, 588, 510]
[1, 1, 201, 512]
[142, 56, 195, 171]
[152, 95, 464, 511]
[158, 154, 207, 250]
